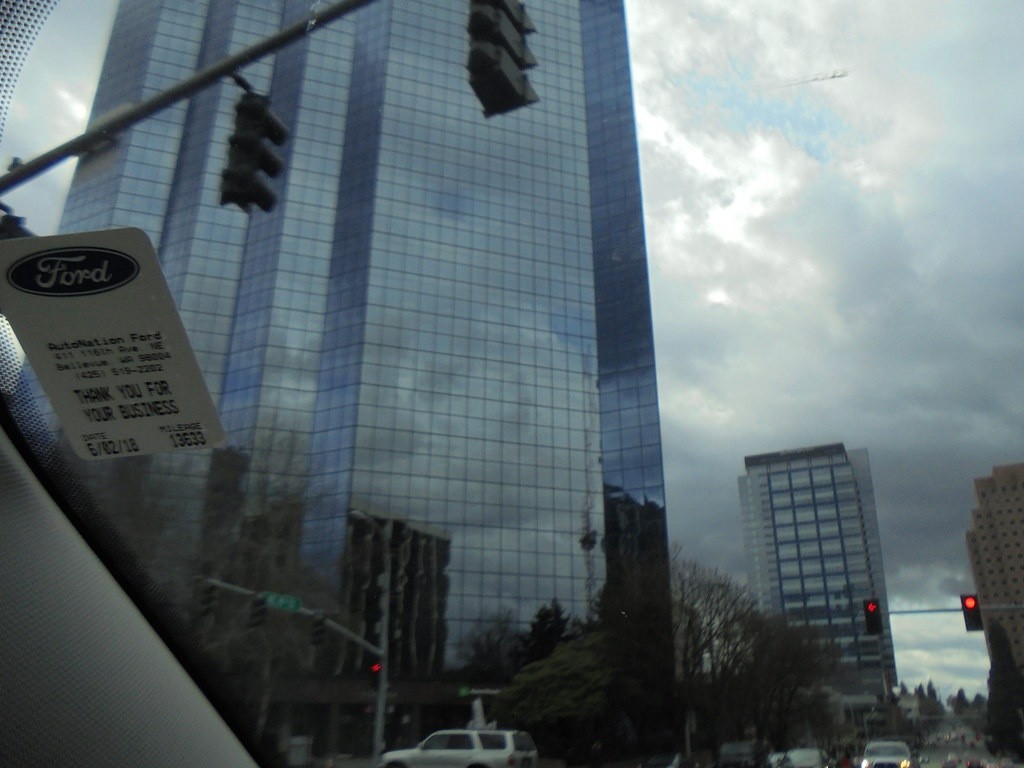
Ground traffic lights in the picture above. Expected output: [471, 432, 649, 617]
[862, 599, 883, 635]
[311, 614, 323, 647]
[367, 661, 381, 692]
[218, 93, 290, 213]
[465, 0, 540, 118]
[200, 584, 216, 617]
[960, 594, 984, 632]
[249, 597, 267, 627]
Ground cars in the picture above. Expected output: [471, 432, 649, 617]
[859, 741, 921, 768]
[942, 733, 985, 768]
[776, 748, 836, 768]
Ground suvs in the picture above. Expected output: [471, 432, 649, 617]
[380, 728, 537, 768]
